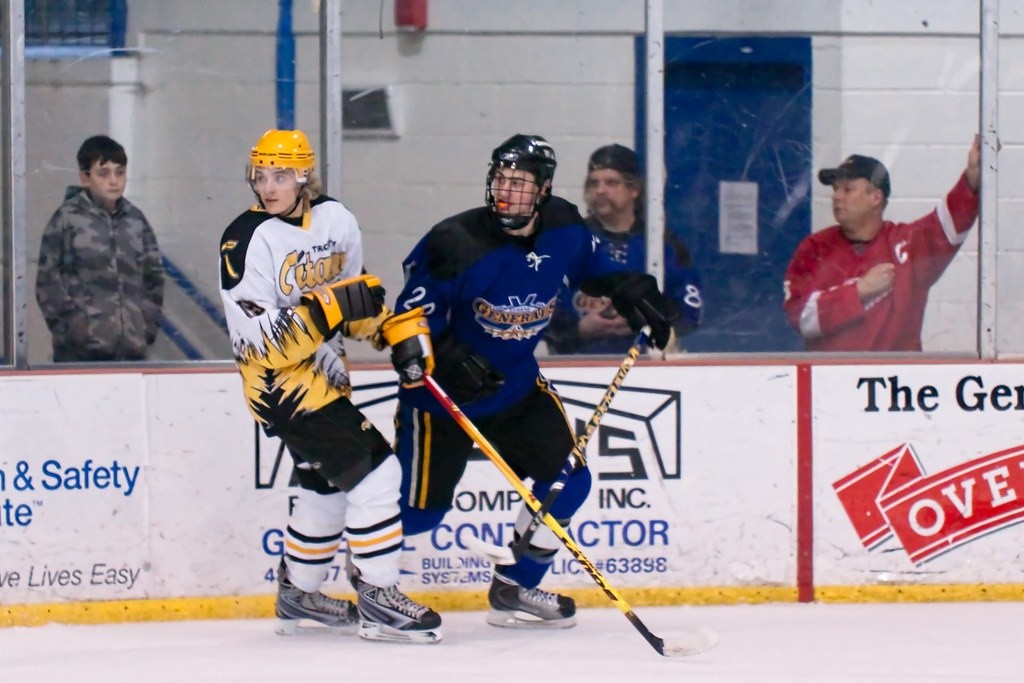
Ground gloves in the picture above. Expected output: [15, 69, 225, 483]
[451, 344, 503, 392]
[619, 297, 670, 350]
[381, 307, 435, 388]
[300, 274, 385, 342]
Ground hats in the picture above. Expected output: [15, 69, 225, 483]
[589, 144, 641, 177]
[819, 154, 890, 198]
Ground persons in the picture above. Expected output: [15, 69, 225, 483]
[784, 133, 1002, 353]
[218, 128, 704, 645]
[36, 135, 166, 363]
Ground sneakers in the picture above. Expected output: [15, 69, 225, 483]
[274, 576, 359, 636]
[489, 570, 578, 628]
[351, 567, 365, 590]
[359, 579, 443, 643]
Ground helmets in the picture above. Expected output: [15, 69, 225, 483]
[246, 129, 316, 184]
[485, 134, 557, 230]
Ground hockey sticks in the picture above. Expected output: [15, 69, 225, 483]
[421, 374, 720, 659]
[465, 325, 652, 565]
